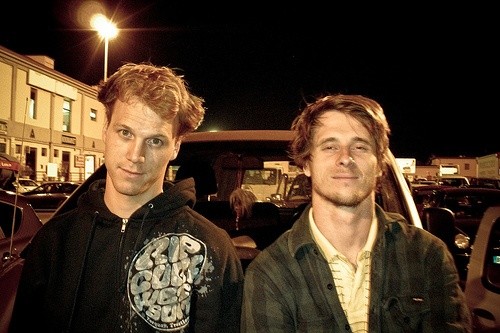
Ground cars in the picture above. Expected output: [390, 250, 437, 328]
[0, 187, 45, 333]
[45, 129, 424, 272]
[14, 182, 81, 210]
[12, 179, 44, 194]
[207, 167, 500, 297]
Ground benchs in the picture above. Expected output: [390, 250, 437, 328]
[194, 199, 309, 250]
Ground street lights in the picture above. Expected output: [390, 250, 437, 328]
[92, 14, 119, 82]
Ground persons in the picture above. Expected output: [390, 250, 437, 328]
[10, 63, 244, 333]
[241, 95, 474, 333]
